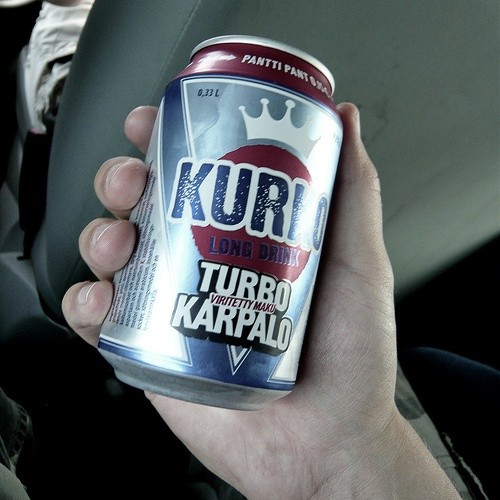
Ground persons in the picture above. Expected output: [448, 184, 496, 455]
[62, 102, 464, 500]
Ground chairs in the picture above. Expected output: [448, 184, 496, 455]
[0, 0, 500, 342]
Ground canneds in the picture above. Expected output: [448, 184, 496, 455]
[95, 34, 343, 411]
